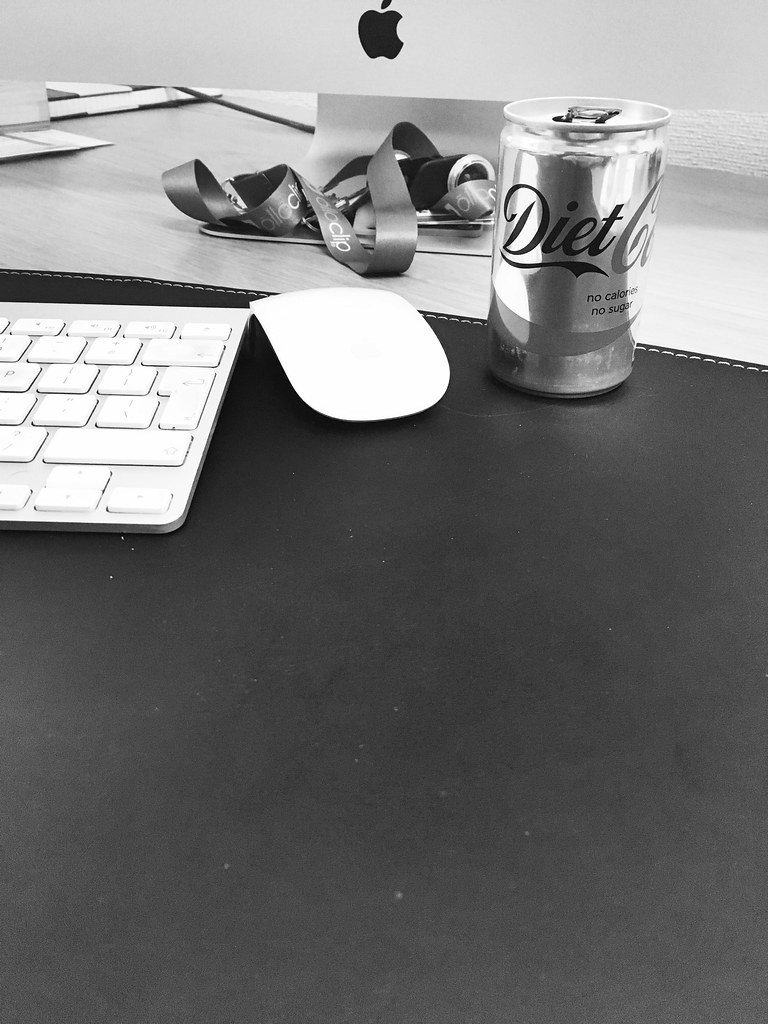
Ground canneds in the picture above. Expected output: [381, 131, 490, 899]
[482, 98, 674, 401]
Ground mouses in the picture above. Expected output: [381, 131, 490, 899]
[252, 286, 451, 422]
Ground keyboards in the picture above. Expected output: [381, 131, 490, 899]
[0, 302, 253, 533]
